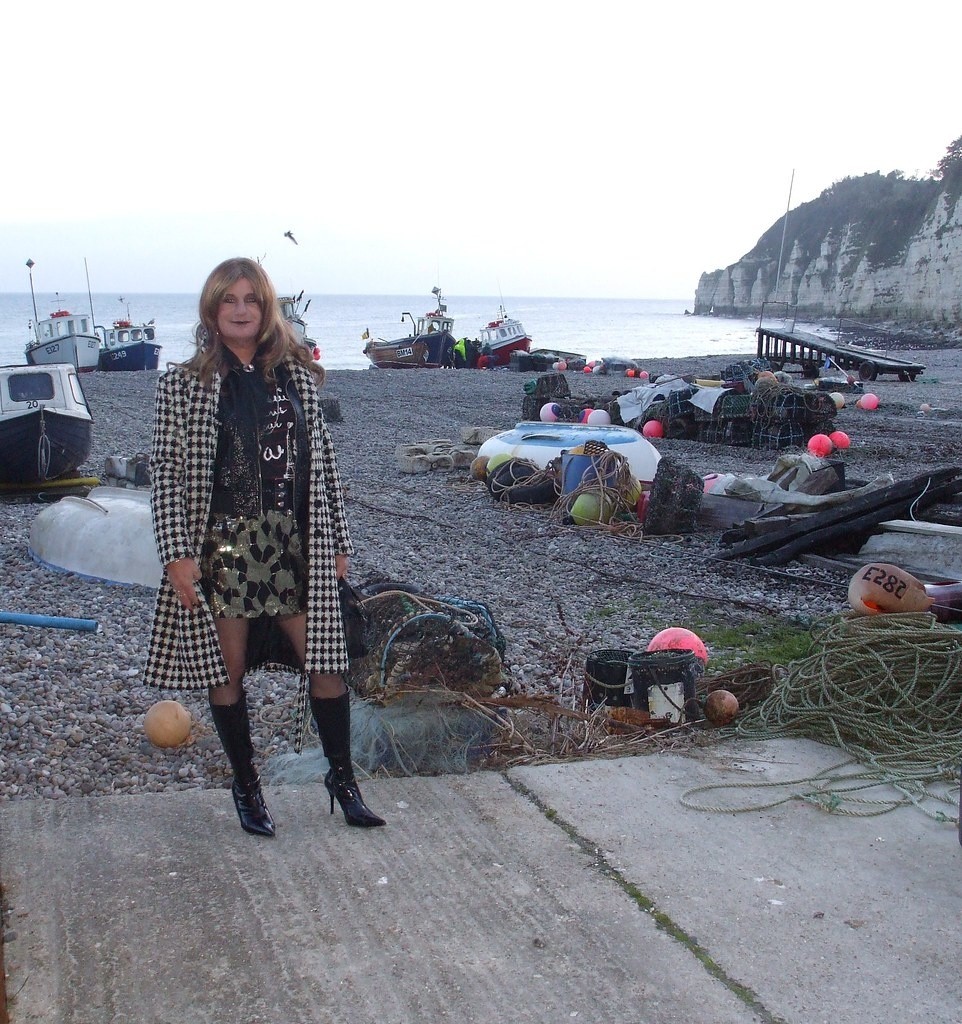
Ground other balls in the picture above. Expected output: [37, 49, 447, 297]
[145, 700, 192, 748]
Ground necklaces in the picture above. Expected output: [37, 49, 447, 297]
[225, 342, 255, 372]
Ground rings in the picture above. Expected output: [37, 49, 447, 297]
[179, 594, 186, 598]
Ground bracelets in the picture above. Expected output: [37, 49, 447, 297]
[168, 558, 183, 565]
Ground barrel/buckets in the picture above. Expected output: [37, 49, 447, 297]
[628, 649, 700, 725]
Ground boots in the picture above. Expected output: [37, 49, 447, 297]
[307, 684, 387, 827]
[209, 691, 276, 838]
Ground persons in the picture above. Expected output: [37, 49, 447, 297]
[142, 258, 387, 839]
[480, 343, 493, 356]
[447, 337, 477, 369]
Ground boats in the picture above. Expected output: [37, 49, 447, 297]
[21, 270, 102, 374]
[0, 361, 101, 497]
[478, 420, 661, 492]
[362, 286, 587, 373]
[95, 299, 162, 372]
[27, 482, 161, 593]
[275, 289, 321, 362]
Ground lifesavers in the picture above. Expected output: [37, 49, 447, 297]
[49, 311, 71, 318]
[113, 324, 130, 327]
[487, 458, 534, 494]
[504, 479, 559, 504]
[489, 324, 499, 327]
[426, 312, 440, 316]
[112, 321, 130, 324]
[488, 321, 502, 324]
[490, 464, 539, 502]
